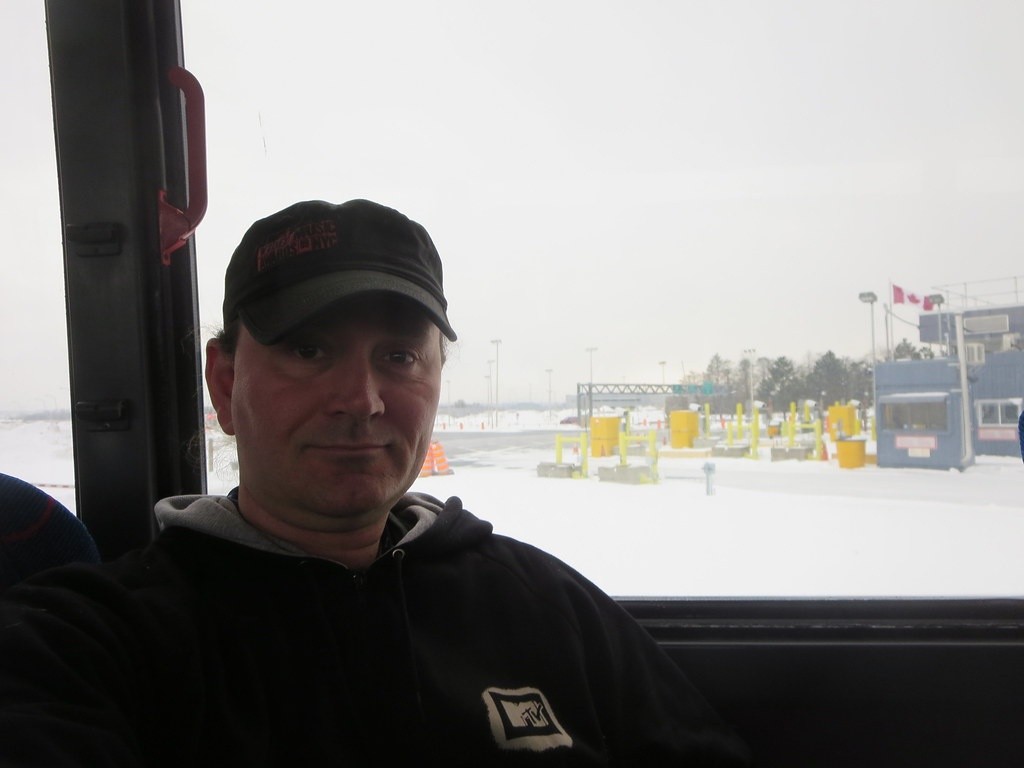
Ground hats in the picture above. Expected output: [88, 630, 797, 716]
[223, 198, 457, 344]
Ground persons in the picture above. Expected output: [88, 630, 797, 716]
[1, 198, 754, 768]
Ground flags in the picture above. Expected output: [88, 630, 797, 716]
[889, 282, 934, 312]
